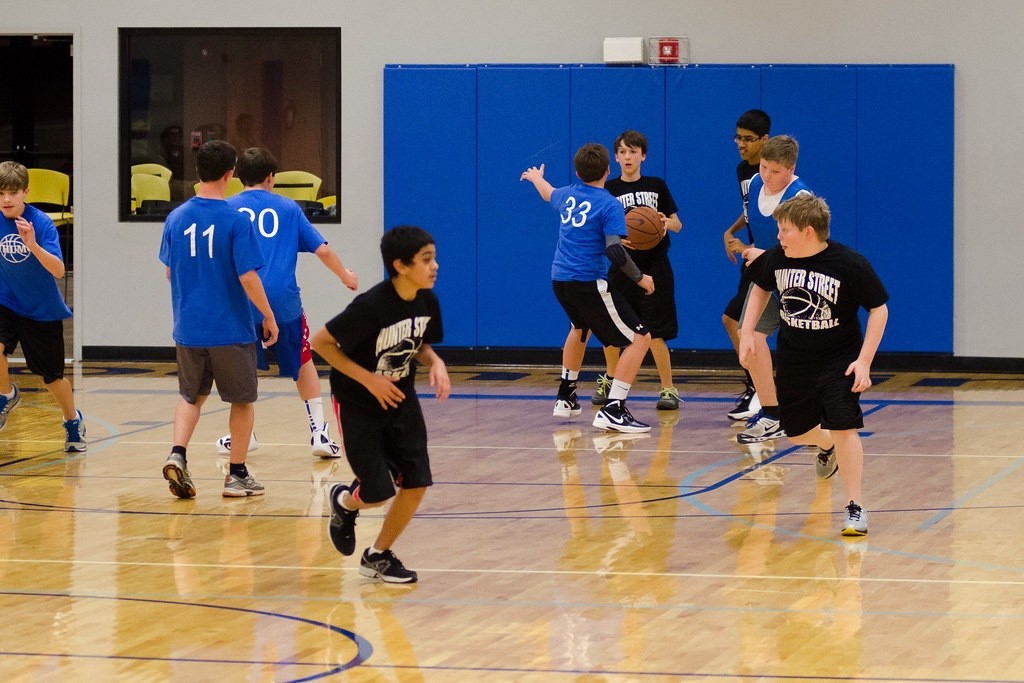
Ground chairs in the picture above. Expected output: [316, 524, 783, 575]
[22, 167, 73, 303]
[131, 162, 176, 212]
[194, 172, 335, 208]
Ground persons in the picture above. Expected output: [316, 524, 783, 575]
[311, 225, 451, 584]
[519, 143, 655, 435]
[215, 147, 358, 458]
[162, 140, 280, 498]
[590, 129, 684, 411]
[723, 109, 814, 444]
[739, 195, 888, 533]
[0, 162, 88, 453]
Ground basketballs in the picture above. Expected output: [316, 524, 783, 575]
[624, 207, 665, 250]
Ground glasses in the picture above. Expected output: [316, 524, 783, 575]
[732, 134, 760, 145]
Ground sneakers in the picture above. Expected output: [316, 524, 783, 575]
[553, 428, 584, 452]
[590, 373, 613, 404]
[728, 380, 762, 420]
[360, 582, 417, 610]
[658, 408, 680, 427]
[0, 383, 21, 430]
[656, 386, 680, 409]
[224, 496, 267, 520]
[64, 451, 87, 489]
[215, 431, 259, 454]
[328, 483, 361, 556]
[737, 409, 787, 443]
[553, 383, 582, 417]
[358, 546, 418, 584]
[311, 455, 339, 496]
[215, 454, 258, 479]
[162, 452, 196, 498]
[311, 424, 341, 458]
[222, 473, 265, 497]
[842, 533, 869, 568]
[593, 433, 652, 462]
[730, 434, 777, 463]
[592, 407, 651, 433]
[815, 446, 838, 480]
[741, 464, 791, 487]
[841, 499, 868, 536]
[62, 409, 87, 453]
